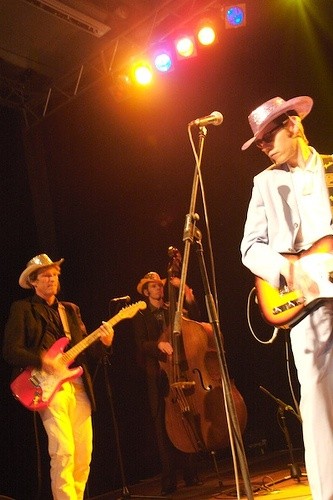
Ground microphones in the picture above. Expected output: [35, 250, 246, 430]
[189, 111, 224, 127]
[110, 296, 131, 303]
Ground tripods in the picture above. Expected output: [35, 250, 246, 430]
[254, 386, 309, 494]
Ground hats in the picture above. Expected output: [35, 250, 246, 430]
[18, 253, 64, 288]
[137, 272, 167, 294]
[241, 96, 314, 150]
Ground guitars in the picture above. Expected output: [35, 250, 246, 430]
[254, 234, 333, 330]
[9, 301, 147, 412]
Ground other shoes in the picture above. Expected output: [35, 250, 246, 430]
[161, 487, 176, 495]
[185, 479, 204, 485]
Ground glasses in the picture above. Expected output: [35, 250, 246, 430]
[254, 123, 283, 148]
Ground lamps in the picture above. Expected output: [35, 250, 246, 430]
[106, 3, 246, 104]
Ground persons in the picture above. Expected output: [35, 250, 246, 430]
[240, 94, 333, 499]
[7, 253, 114, 500]
[134, 271, 205, 496]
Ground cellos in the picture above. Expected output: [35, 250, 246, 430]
[156, 247, 247, 489]
